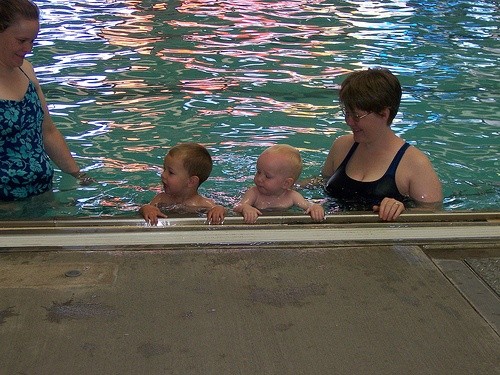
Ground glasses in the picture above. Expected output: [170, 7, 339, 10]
[341, 106, 372, 122]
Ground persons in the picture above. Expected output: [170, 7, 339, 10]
[0, 0, 98, 214]
[233, 144, 325, 225]
[291, 68, 442, 221]
[139, 143, 228, 225]
